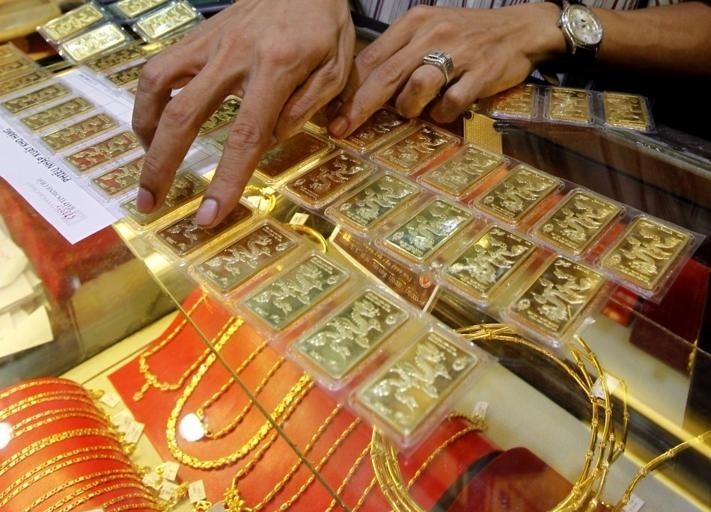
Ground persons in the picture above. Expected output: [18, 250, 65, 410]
[132, 1, 711, 229]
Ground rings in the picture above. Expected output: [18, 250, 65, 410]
[423, 47, 455, 87]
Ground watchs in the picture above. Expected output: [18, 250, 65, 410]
[545, 1, 605, 89]
